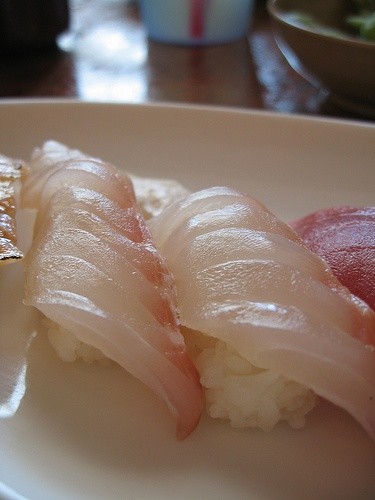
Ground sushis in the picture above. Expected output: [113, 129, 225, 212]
[16, 140, 375, 439]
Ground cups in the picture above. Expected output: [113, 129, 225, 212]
[142, 1, 254, 45]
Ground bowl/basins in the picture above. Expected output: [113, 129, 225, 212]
[265, 0, 375, 119]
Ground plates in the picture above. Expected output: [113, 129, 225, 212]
[1, 98, 375, 500]
